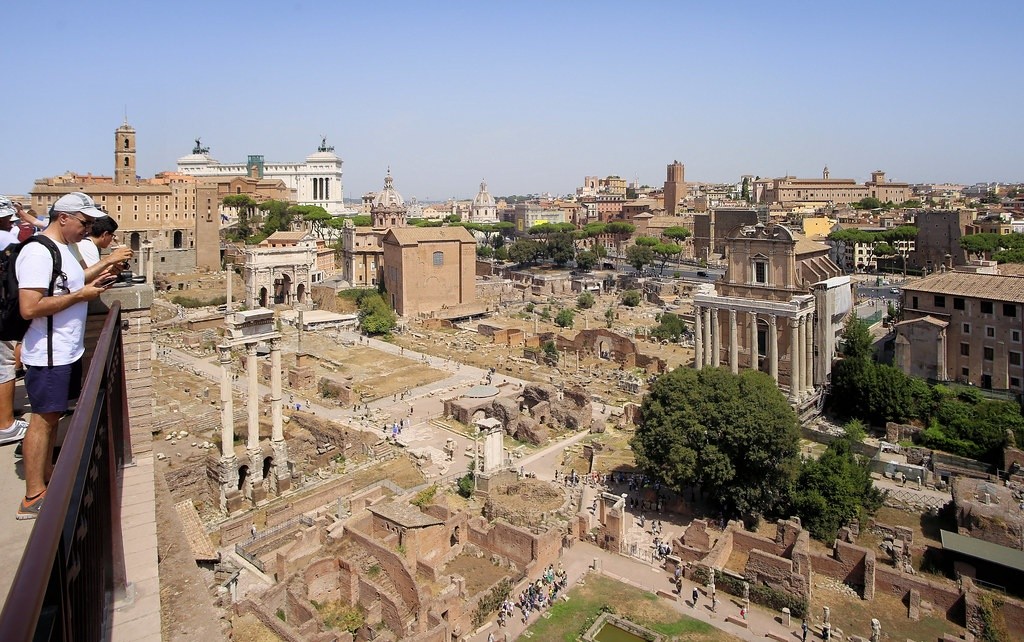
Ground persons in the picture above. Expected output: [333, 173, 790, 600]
[641, 514, 662, 534]
[693, 587, 698, 605]
[712, 592, 716, 608]
[674, 566, 682, 594]
[555, 470, 661, 494]
[384, 418, 403, 434]
[902, 475, 906, 487]
[630, 498, 646, 512]
[349, 417, 351, 423]
[917, 476, 921, 490]
[741, 605, 748, 619]
[593, 497, 598, 514]
[488, 631, 494, 642]
[654, 537, 672, 557]
[498, 564, 568, 628]
[0, 192, 134, 520]
[297, 407, 299, 410]
[159, 344, 171, 355]
[801, 618, 808, 642]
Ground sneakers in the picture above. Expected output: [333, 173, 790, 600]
[0, 420, 30, 444]
[16, 490, 48, 519]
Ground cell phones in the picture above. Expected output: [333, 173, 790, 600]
[99, 279, 117, 289]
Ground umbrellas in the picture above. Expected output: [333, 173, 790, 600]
[296, 404, 301, 407]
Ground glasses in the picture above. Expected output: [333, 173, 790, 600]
[108, 233, 116, 239]
[69, 214, 93, 228]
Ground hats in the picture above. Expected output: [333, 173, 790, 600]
[52, 192, 107, 219]
[0, 196, 18, 217]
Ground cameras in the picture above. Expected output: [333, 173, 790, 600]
[121, 259, 130, 270]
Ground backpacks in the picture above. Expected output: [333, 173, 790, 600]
[0, 234, 62, 341]
